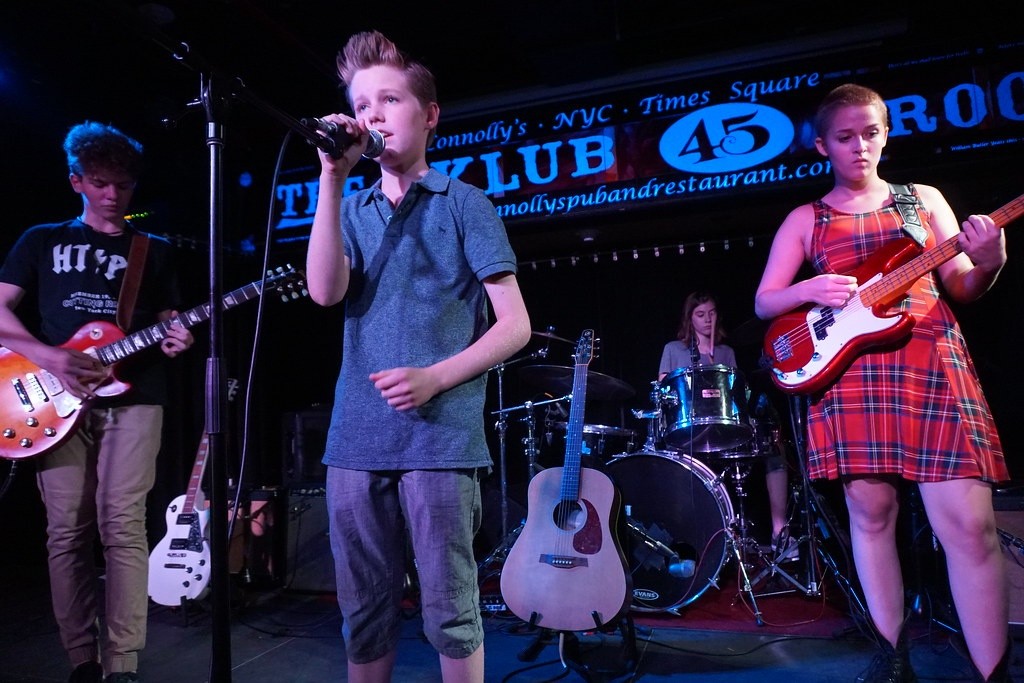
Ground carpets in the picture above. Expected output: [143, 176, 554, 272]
[398, 573, 857, 638]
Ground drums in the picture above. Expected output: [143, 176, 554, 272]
[539, 420, 635, 469]
[653, 361, 754, 452]
[594, 449, 737, 615]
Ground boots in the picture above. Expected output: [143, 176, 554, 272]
[948, 632, 1016, 683]
[853, 606, 917, 683]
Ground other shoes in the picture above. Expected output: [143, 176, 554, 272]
[67, 661, 103, 683]
[767, 567, 804, 596]
[106, 672, 144, 683]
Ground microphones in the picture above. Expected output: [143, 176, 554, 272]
[545, 393, 570, 420]
[668, 559, 696, 578]
[690, 325, 701, 360]
[301, 118, 386, 159]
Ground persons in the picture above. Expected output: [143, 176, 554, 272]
[306, 32, 532, 683]
[0, 122, 193, 683]
[659, 291, 800, 558]
[754, 85, 1012, 682]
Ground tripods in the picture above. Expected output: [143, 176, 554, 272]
[478, 327, 963, 639]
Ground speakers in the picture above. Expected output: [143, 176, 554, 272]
[279, 410, 331, 490]
[285, 482, 412, 596]
[992, 495, 1024, 638]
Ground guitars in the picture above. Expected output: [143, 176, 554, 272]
[147, 378, 240, 608]
[0, 262, 307, 463]
[763, 193, 1024, 395]
[501, 330, 635, 633]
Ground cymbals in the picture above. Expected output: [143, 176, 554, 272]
[531, 331, 579, 346]
[517, 364, 634, 405]
